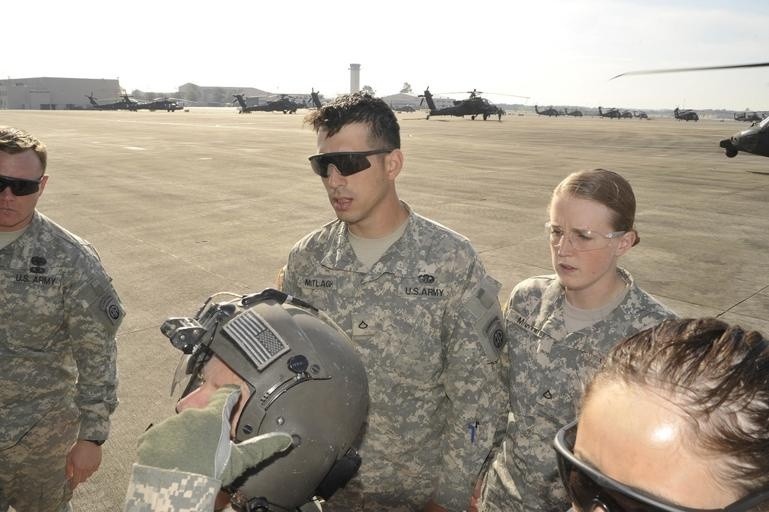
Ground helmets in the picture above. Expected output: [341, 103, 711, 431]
[160, 284, 371, 510]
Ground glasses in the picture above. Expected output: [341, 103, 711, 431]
[0, 172, 45, 195]
[553, 420, 769, 512]
[545, 223, 625, 253]
[307, 147, 388, 176]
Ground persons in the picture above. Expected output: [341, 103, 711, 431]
[276, 89, 507, 511]
[550, 316, 769, 511]
[480, 167, 670, 511]
[0, 121, 127, 511]
[115, 290, 371, 511]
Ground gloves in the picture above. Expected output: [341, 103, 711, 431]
[126, 384, 293, 509]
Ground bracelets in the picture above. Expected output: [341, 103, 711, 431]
[84, 438, 106, 446]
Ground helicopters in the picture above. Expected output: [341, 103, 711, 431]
[83, 91, 198, 113]
[609, 62, 769, 158]
